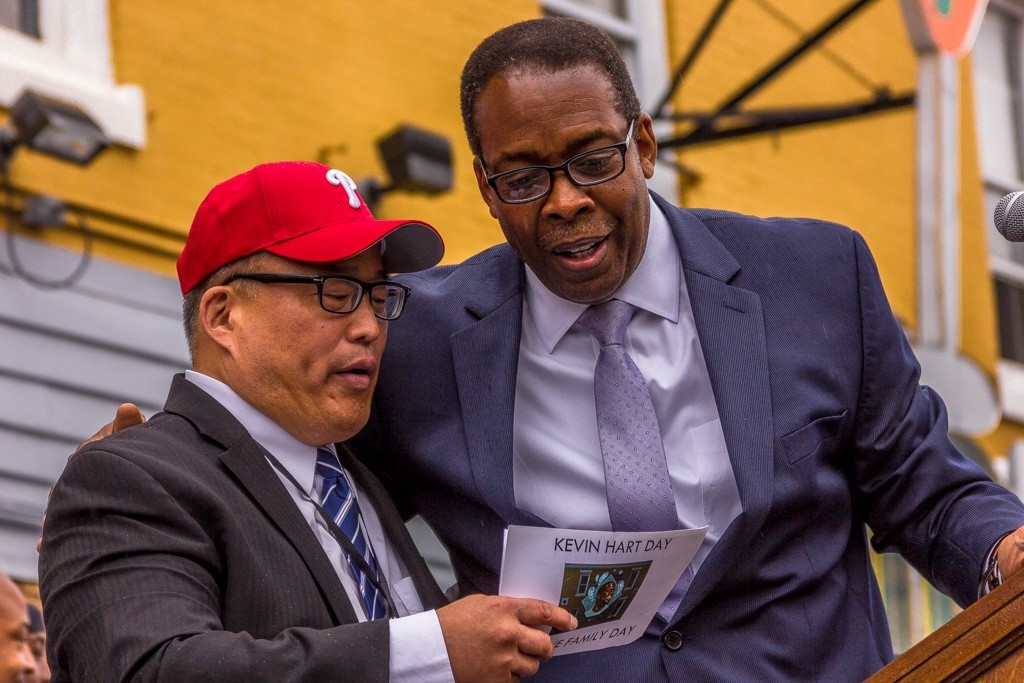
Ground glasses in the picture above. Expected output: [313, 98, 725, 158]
[220, 273, 413, 322]
[477, 118, 640, 203]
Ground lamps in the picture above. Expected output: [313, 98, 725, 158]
[0, 90, 109, 171]
[352, 125, 454, 205]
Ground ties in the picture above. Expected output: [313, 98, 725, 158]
[577, 300, 696, 624]
[309, 441, 394, 622]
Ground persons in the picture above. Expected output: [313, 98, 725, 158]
[34, 17, 1024, 682]
[39, 160, 578, 682]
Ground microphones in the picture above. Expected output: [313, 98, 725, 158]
[993, 190, 1024, 243]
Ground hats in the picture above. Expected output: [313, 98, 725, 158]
[175, 161, 444, 295]
